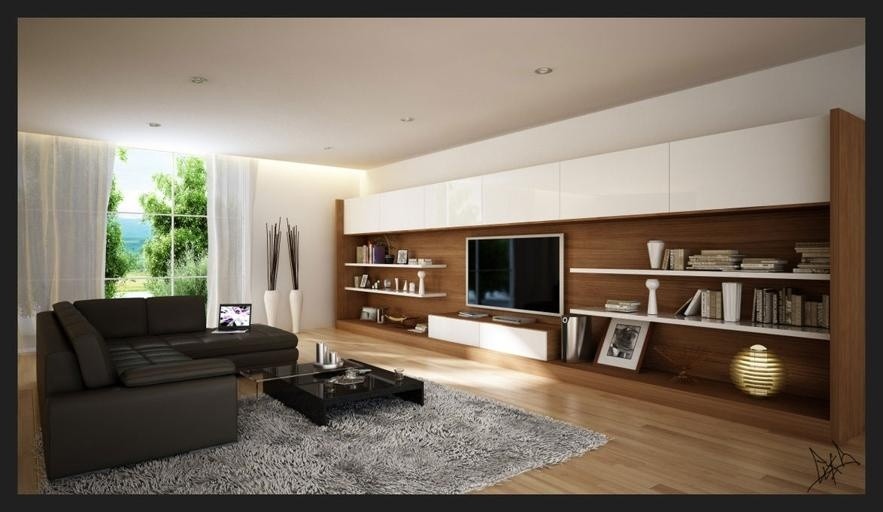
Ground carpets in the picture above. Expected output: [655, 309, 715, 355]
[32, 376, 617, 496]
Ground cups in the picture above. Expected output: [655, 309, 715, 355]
[314, 341, 344, 369]
[393, 368, 407, 382]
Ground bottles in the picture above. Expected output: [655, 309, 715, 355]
[377, 304, 385, 325]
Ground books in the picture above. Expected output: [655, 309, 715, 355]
[604, 241, 831, 329]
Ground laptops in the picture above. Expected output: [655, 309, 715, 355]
[211, 303, 252, 334]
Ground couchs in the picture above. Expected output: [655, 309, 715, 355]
[36, 295, 298, 481]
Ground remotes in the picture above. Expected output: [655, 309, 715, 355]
[314, 368, 347, 378]
[358, 369, 372, 375]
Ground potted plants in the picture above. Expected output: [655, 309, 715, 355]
[286, 217, 303, 334]
[264, 217, 282, 329]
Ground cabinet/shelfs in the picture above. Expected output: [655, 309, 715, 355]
[334, 108, 866, 446]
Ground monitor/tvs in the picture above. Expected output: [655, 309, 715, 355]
[465, 232, 565, 318]
